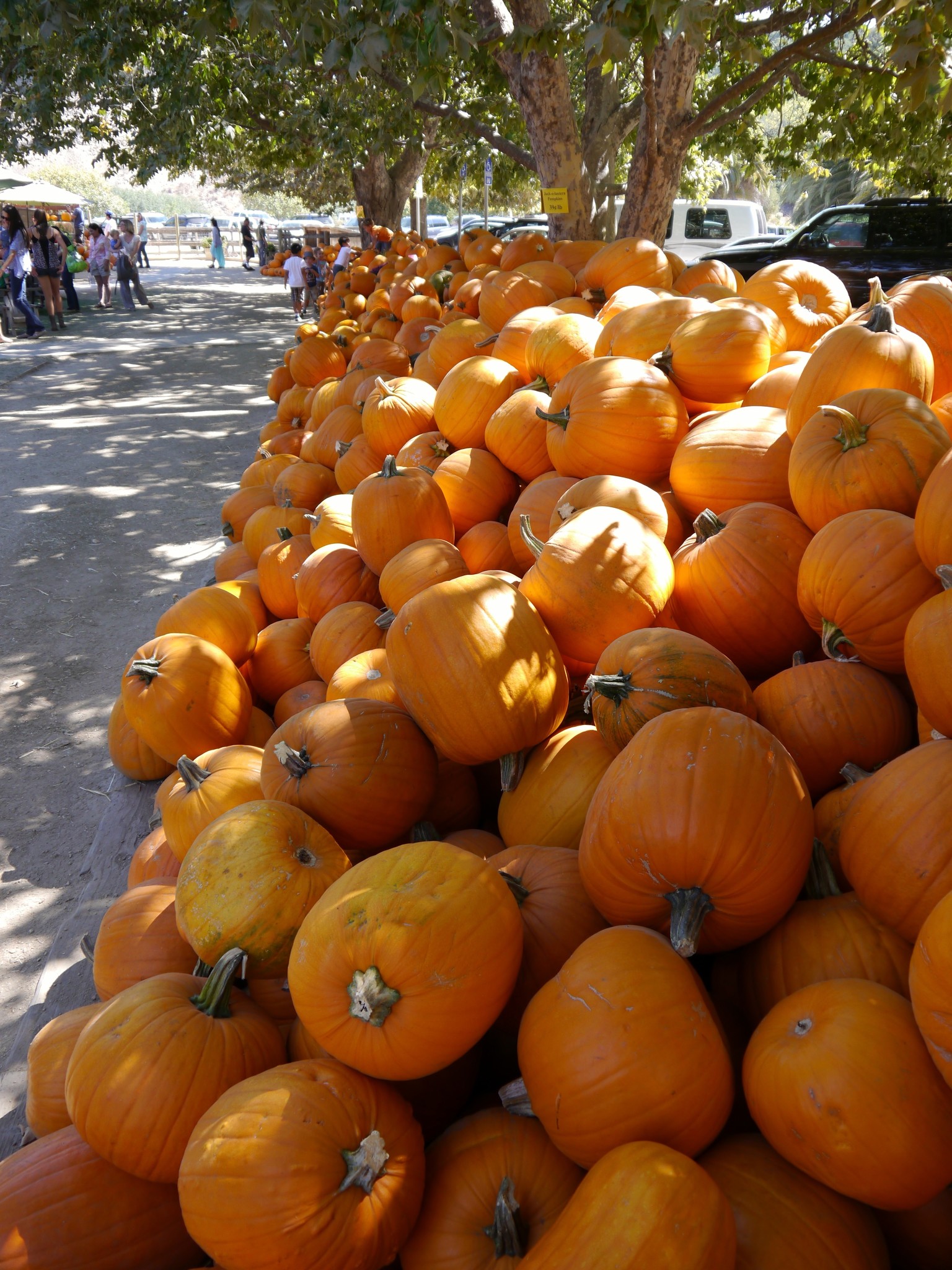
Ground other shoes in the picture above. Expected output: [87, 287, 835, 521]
[130, 307, 136, 311]
[147, 303, 154, 309]
[16, 333, 31, 338]
[28, 329, 46, 340]
[145, 265, 150, 268]
[0, 337, 13, 343]
[138, 265, 143, 268]
[247, 266, 255, 271]
[76, 309, 79, 311]
[65, 308, 75, 311]
[241, 263, 246, 268]
[294, 309, 318, 321]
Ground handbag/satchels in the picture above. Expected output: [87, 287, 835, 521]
[61, 244, 88, 273]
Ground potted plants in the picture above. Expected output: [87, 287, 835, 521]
[254, 241, 279, 266]
[200, 235, 228, 260]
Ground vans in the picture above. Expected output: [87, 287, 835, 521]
[278, 212, 336, 240]
[589, 198, 767, 266]
[769, 226, 795, 236]
[400, 214, 450, 236]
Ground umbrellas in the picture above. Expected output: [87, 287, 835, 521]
[0, 166, 99, 228]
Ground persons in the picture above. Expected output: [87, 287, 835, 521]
[134, 212, 150, 269]
[23, 208, 68, 332]
[0, 204, 164, 344]
[208, 217, 225, 269]
[362, 218, 394, 255]
[254, 219, 268, 267]
[86, 223, 112, 308]
[282, 243, 333, 321]
[240, 217, 258, 271]
[333, 236, 362, 288]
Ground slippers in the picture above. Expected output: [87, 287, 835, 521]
[93, 304, 104, 307]
[105, 304, 112, 307]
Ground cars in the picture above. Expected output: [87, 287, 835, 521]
[341, 214, 360, 228]
[435, 213, 550, 248]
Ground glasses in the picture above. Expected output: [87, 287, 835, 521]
[1, 213, 8, 219]
[119, 224, 126, 226]
[137, 216, 141, 217]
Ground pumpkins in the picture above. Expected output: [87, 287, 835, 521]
[50, 215, 58, 221]
[61, 211, 71, 222]
[1, 229, 952, 1270]
[45, 212, 50, 221]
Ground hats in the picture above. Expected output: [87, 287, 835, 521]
[105, 210, 113, 215]
[303, 252, 315, 261]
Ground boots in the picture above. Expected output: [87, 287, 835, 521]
[57, 314, 66, 328]
[49, 315, 58, 331]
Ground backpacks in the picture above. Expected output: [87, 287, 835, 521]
[115, 251, 136, 296]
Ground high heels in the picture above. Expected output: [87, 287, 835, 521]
[218, 267, 225, 269]
[209, 265, 214, 268]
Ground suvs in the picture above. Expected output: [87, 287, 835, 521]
[95, 211, 169, 239]
[160, 213, 212, 241]
[203, 209, 282, 239]
[684, 195, 952, 310]
